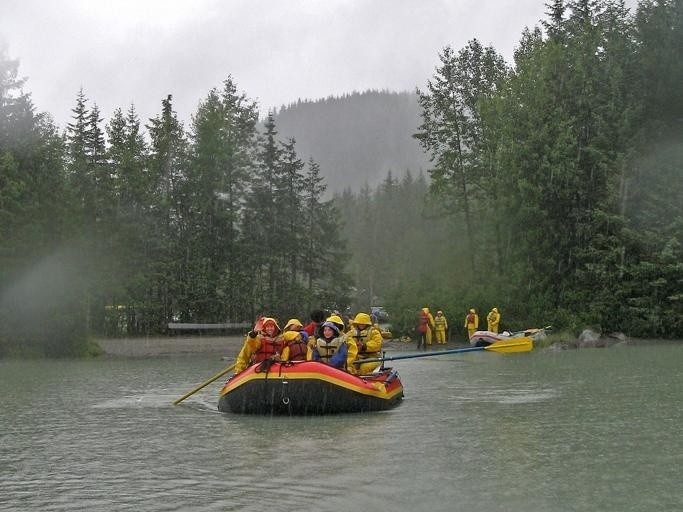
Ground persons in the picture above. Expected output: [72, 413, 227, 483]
[487, 307, 500, 333]
[415, 310, 430, 351]
[463, 308, 479, 338]
[434, 311, 448, 345]
[234, 309, 385, 377]
[421, 307, 436, 346]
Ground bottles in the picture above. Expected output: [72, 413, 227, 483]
[264, 320, 274, 326]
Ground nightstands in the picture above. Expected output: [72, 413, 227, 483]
[352, 336, 533, 367]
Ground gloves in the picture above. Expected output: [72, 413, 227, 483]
[379, 330, 392, 338]
[467, 326, 545, 350]
[216, 359, 406, 415]
